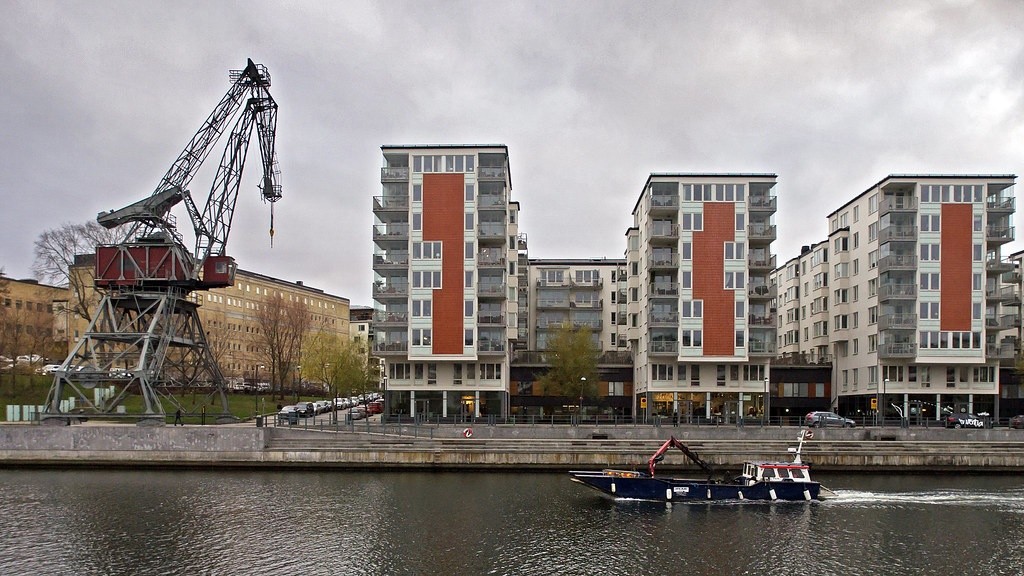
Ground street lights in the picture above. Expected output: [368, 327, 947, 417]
[883, 377, 890, 420]
[292, 364, 301, 405]
[579, 376, 586, 423]
[322, 364, 330, 400]
[763, 377, 769, 426]
[376, 363, 388, 417]
[255, 364, 265, 416]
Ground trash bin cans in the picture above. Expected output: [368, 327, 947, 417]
[30, 412, 41, 426]
[288, 411, 298, 425]
[256, 415, 263, 427]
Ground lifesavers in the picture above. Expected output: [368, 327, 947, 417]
[463, 428, 473, 438]
[806, 430, 814, 439]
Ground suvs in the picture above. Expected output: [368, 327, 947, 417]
[946, 411, 994, 430]
[804, 411, 856, 428]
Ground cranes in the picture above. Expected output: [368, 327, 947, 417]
[40, 57, 282, 426]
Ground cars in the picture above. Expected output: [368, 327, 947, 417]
[1011, 414, 1024, 429]
[0, 353, 270, 392]
[276, 392, 385, 421]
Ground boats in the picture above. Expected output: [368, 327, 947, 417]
[568, 428, 821, 502]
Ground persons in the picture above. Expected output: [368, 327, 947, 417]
[174, 409, 184, 426]
[277, 401, 283, 411]
[673, 408, 679, 427]
